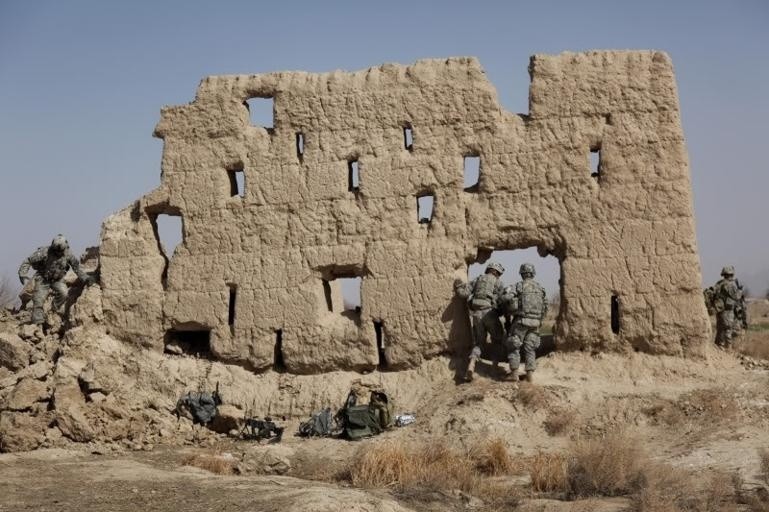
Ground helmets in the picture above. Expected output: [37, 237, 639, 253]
[485, 262, 505, 274]
[519, 263, 534, 275]
[720, 266, 735, 275]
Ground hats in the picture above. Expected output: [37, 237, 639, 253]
[52, 238, 66, 250]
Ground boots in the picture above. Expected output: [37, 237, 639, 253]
[32, 309, 44, 324]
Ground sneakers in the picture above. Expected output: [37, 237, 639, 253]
[466, 365, 534, 383]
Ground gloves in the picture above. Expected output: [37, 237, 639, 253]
[20, 277, 29, 285]
[454, 279, 464, 288]
[87, 277, 95, 286]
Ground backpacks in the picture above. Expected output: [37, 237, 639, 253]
[704, 280, 725, 316]
[299, 389, 391, 440]
[516, 281, 543, 329]
[472, 275, 496, 309]
[176, 390, 214, 422]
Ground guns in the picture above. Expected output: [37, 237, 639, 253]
[733, 279, 749, 332]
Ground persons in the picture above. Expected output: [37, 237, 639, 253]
[17, 231, 98, 326]
[453, 261, 512, 384]
[499, 263, 550, 383]
[710, 265, 748, 355]
